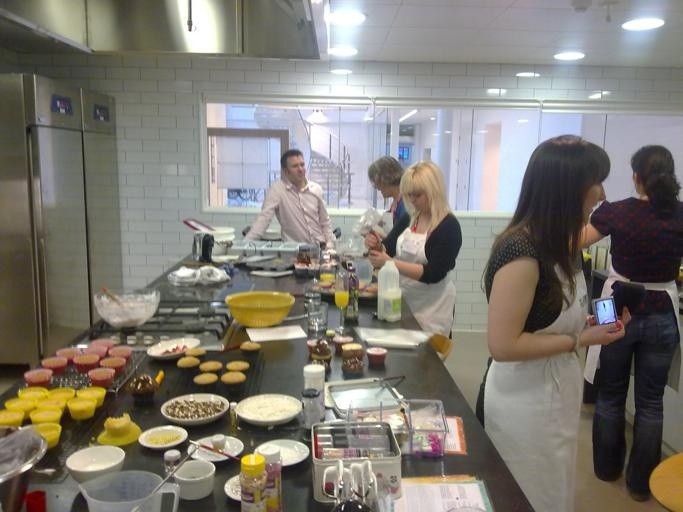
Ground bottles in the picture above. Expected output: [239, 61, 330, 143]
[240, 451, 266, 512]
[228, 400, 237, 436]
[376, 258, 403, 324]
[300, 365, 326, 430]
[257, 444, 281, 512]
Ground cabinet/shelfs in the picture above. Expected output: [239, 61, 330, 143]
[86, 1, 319, 61]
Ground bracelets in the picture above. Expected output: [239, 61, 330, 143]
[568, 331, 579, 351]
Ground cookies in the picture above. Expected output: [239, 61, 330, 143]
[176, 341, 261, 385]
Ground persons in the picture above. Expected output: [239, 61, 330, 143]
[475, 133, 625, 512]
[368, 155, 411, 282]
[364, 160, 462, 363]
[244, 148, 335, 251]
[581, 145, 683, 500]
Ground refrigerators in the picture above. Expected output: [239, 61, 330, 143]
[0, 71, 124, 372]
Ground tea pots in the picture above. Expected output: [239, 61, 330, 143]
[78, 470, 180, 512]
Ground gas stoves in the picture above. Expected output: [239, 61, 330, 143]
[70, 300, 236, 352]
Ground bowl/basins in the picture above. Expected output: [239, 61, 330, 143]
[92, 287, 160, 328]
[65, 445, 126, 482]
[0, 424, 48, 512]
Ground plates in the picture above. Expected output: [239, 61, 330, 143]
[158, 393, 228, 426]
[146, 337, 200, 361]
[222, 473, 239, 502]
[137, 425, 189, 450]
[251, 439, 309, 466]
[235, 393, 303, 427]
[184, 435, 245, 462]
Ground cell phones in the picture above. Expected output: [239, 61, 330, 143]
[591, 296, 618, 326]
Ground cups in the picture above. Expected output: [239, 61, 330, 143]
[297, 242, 320, 263]
[304, 259, 386, 368]
[172, 461, 215, 502]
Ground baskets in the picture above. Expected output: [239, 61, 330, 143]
[224, 290, 298, 330]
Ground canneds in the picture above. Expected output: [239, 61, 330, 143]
[342, 343, 364, 375]
